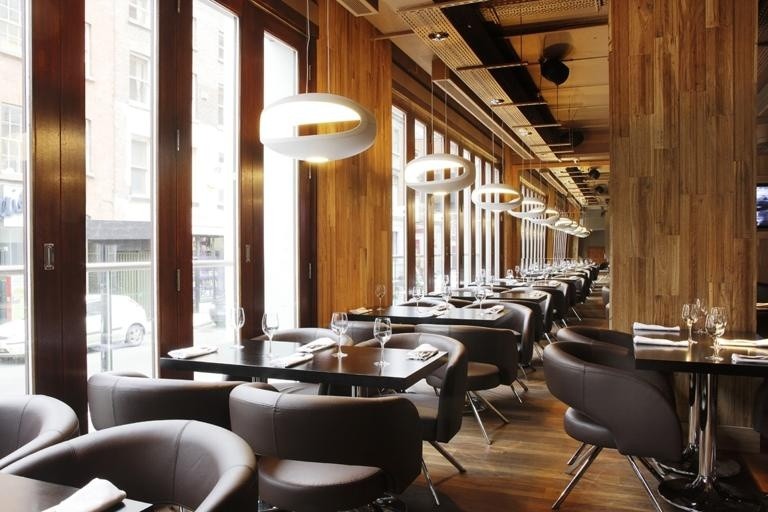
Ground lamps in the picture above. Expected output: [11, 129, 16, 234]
[258, 0, 377, 164]
[404, 31, 592, 238]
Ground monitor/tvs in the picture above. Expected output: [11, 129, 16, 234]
[755, 182, 768, 230]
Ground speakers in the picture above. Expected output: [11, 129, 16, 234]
[560, 130, 585, 149]
[589, 169, 600, 181]
[596, 186, 603, 194]
[538, 55, 570, 85]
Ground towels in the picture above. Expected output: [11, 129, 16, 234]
[715, 336, 768, 348]
[632, 334, 699, 347]
[730, 352, 768, 365]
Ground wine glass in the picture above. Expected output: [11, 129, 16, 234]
[412, 286, 425, 312]
[681, 297, 727, 362]
[473, 288, 486, 314]
[329, 312, 349, 358]
[442, 258, 592, 292]
[234, 307, 247, 349]
[374, 284, 387, 310]
[372, 318, 392, 367]
[261, 312, 279, 356]
[441, 286, 453, 314]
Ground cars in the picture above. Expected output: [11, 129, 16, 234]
[211, 303, 226, 325]
[0, 294, 148, 358]
